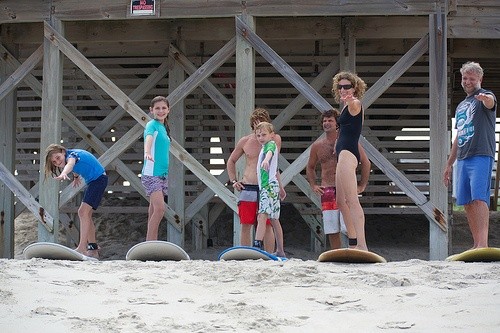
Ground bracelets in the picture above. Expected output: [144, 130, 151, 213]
[145, 152, 151, 154]
[74, 176, 80, 178]
[231, 180, 238, 185]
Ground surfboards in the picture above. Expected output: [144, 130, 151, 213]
[21, 242, 98, 260]
[124, 239, 192, 262]
[316, 247, 387, 264]
[445, 247, 500, 263]
[217, 246, 288, 261]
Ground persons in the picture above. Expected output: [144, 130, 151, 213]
[141, 97, 172, 241]
[306, 110, 370, 251]
[227, 108, 281, 253]
[443, 62, 497, 249]
[44, 144, 108, 258]
[333, 72, 366, 252]
[253, 122, 286, 257]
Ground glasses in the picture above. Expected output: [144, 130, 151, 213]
[337, 84, 354, 89]
